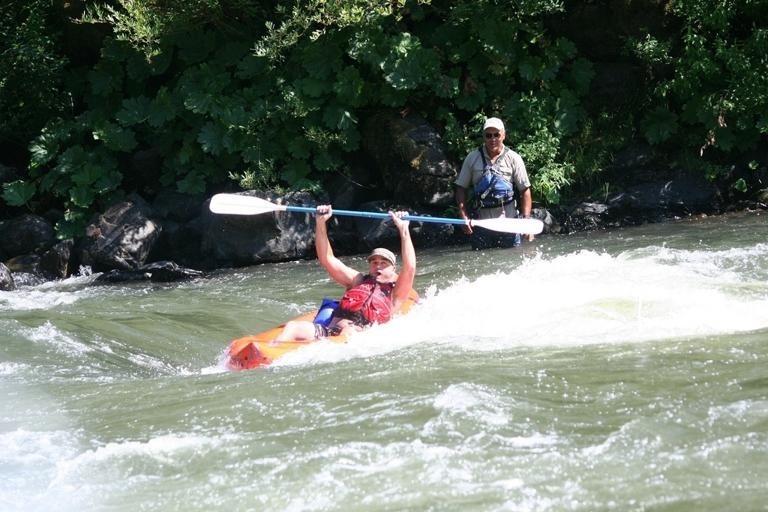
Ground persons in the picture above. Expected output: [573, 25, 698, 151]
[270, 203, 420, 349]
[452, 116, 536, 247]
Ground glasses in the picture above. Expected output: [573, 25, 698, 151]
[485, 132, 501, 138]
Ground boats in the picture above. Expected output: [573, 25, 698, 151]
[223, 271, 421, 373]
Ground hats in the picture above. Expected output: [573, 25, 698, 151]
[367, 248, 396, 266]
[483, 117, 505, 131]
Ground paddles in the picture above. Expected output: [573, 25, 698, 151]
[209, 193, 543, 235]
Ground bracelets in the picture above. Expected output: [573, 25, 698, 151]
[522, 214, 530, 218]
